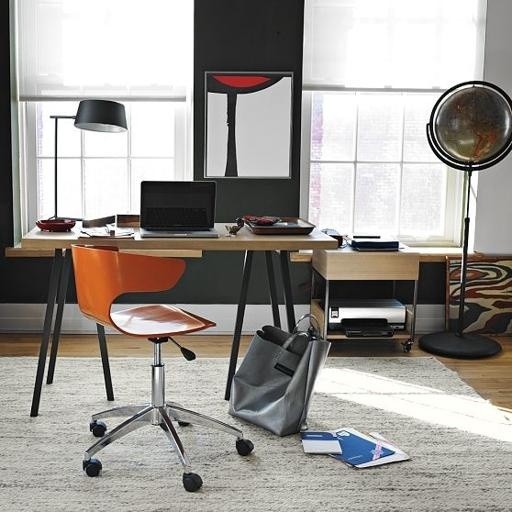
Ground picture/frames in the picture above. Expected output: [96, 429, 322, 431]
[5, 219, 339, 417]
[309, 241, 420, 353]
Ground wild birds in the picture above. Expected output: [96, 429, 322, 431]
[140, 180, 218, 237]
[245, 215, 316, 235]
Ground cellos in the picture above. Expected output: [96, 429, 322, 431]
[328, 298, 406, 336]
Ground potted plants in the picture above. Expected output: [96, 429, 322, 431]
[202, 69, 294, 180]
[446, 255, 512, 338]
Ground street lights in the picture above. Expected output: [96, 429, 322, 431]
[227, 325, 332, 437]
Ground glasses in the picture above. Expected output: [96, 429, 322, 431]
[35, 99, 128, 233]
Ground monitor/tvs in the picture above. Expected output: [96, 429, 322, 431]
[79, 226, 135, 240]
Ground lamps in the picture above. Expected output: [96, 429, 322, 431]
[70, 243, 254, 492]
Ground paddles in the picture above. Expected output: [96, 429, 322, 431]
[0, 355, 512, 512]
